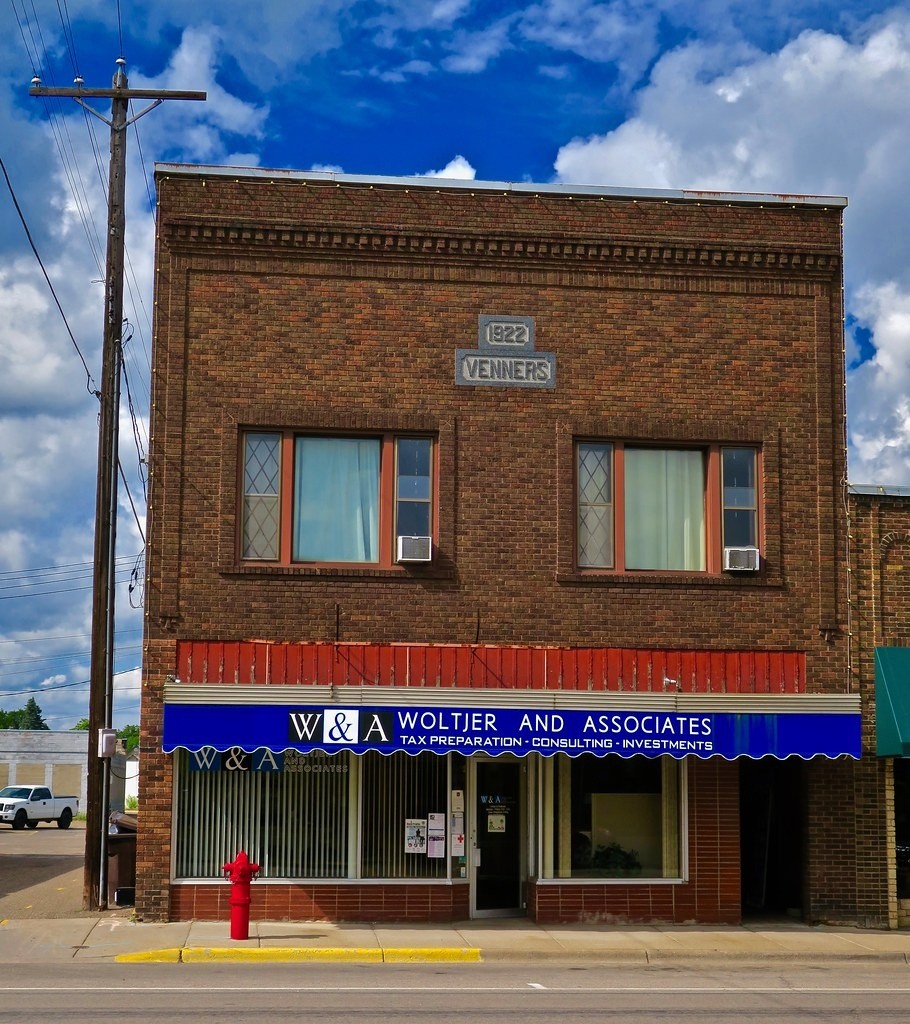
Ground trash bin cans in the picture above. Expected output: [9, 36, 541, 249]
[106, 811, 137, 910]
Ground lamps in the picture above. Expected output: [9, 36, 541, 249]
[664, 676, 676, 687]
[168, 674, 181, 684]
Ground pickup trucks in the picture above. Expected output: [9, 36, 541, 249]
[0, 785, 80, 830]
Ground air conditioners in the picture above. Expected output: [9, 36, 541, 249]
[724, 548, 759, 570]
[396, 536, 431, 563]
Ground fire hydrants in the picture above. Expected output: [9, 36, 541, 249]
[221, 851, 260, 940]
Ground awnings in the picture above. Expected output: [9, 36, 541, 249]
[162, 682, 862, 762]
[874, 644, 910, 760]
